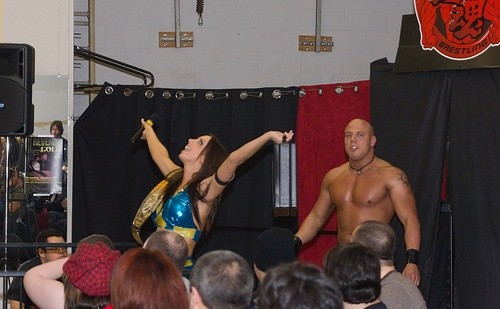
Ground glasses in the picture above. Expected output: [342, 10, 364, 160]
[45, 247, 67, 252]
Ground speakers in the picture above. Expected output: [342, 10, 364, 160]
[0, 43, 35, 136]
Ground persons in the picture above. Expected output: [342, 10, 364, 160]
[31, 151, 52, 176]
[289, 119, 421, 289]
[129, 117, 294, 276]
[0, 220, 429, 309]
[49, 120, 68, 185]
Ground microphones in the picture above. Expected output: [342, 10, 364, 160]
[128, 112, 160, 145]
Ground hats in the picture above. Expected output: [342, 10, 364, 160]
[63, 235, 122, 296]
